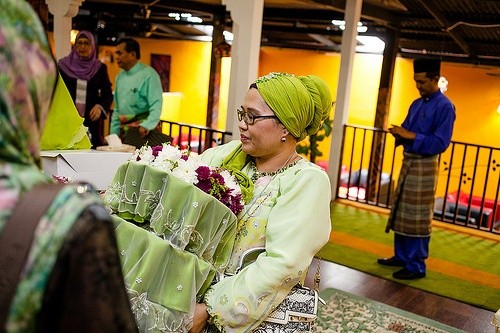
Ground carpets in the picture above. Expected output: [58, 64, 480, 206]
[314, 288, 472, 333]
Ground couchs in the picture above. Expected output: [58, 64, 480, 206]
[434, 189, 500, 229]
[343, 169, 394, 205]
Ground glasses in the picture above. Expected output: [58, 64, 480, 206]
[78, 41, 89, 46]
[237, 109, 276, 125]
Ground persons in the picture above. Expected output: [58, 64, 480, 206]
[57, 31, 113, 151]
[0, 0, 139, 333]
[109, 38, 163, 150]
[378, 56, 456, 280]
[185, 73, 331, 333]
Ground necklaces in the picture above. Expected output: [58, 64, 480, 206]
[235, 152, 299, 239]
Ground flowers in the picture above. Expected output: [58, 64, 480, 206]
[129, 140, 245, 216]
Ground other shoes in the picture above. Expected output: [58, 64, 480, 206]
[378, 257, 404, 267]
[392, 268, 425, 280]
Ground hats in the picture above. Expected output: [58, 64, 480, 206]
[414, 58, 441, 73]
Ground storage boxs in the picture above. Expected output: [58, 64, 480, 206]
[39, 150, 134, 191]
